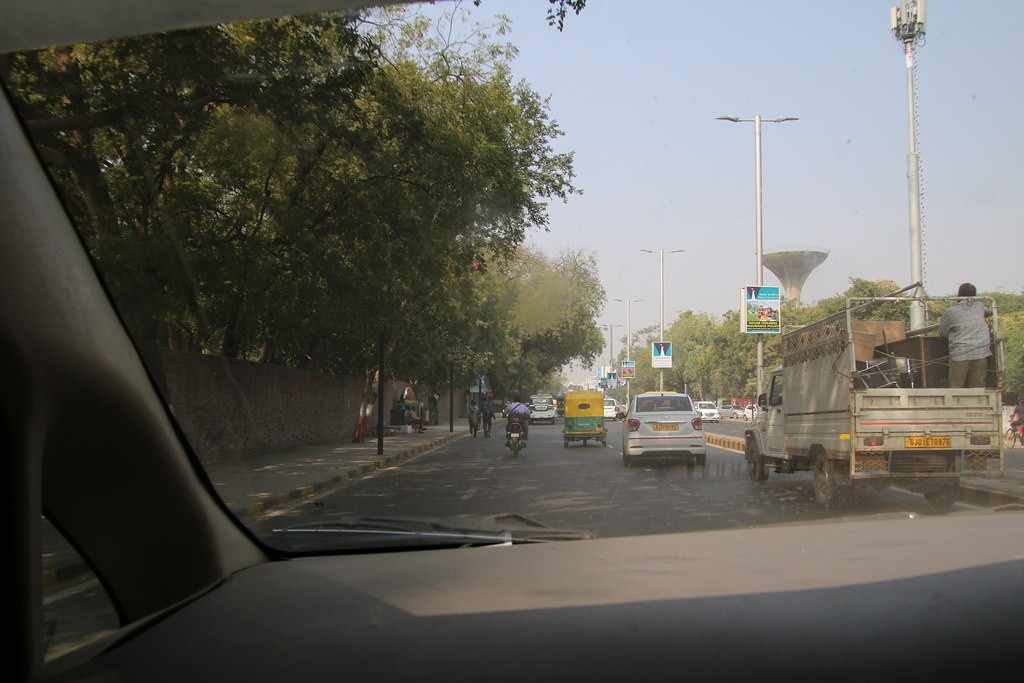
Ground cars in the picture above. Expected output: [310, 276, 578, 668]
[604, 398, 618, 421]
[694, 401, 721, 423]
[718, 404, 745, 419]
[616, 392, 707, 466]
[743, 404, 758, 421]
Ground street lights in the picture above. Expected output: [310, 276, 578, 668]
[716, 113, 799, 418]
[639, 249, 685, 395]
[615, 298, 644, 410]
[602, 324, 623, 390]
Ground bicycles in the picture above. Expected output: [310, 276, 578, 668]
[1004, 419, 1024, 450]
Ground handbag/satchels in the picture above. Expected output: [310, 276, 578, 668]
[508, 410, 525, 424]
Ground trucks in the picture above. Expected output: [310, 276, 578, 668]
[739, 282, 1008, 512]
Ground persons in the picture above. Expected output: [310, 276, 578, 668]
[404, 405, 427, 434]
[1009, 400, 1024, 443]
[560, 401, 564, 407]
[502, 396, 530, 446]
[645, 402, 654, 411]
[699, 405, 702, 408]
[939, 283, 992, 388]
[468, 397, 495, 438]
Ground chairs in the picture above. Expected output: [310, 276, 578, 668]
[866, 357, 914, 388]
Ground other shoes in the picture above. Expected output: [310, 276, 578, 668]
[421, 428, 427, 430]
[418, 431, 423, 433]
[505, 441, 511, 446]
[474, 434, 476, 437]
[488, 432, 490, 437]
[470, 431, 473, 433]
[484, 435, 487, 437]
[521, 442, 526, 447]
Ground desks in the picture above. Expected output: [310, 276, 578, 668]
[874, 337, 950, 388]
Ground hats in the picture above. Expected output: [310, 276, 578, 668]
[959, 283, 976, 296]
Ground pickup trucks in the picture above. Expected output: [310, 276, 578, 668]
[527, 394, 557, 425]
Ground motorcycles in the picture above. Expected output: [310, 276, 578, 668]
[562, 391, 609, 450]
[502, 410, 528, 458]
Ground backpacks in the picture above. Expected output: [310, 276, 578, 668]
[482, 402, 492, 418]
[468, 405, 481, 425]
